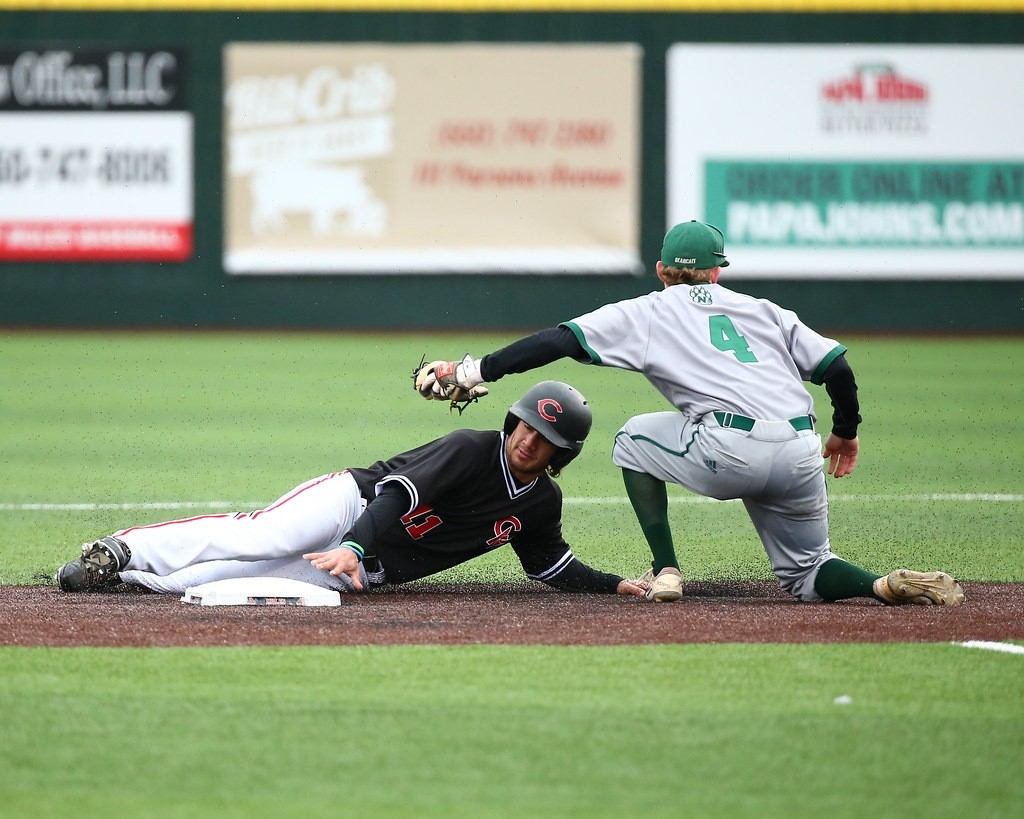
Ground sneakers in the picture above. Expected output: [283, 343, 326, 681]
[877, 568, 965, 608]
[635, 567, 684, 602]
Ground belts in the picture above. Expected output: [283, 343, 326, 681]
[716, 412, 813, 433]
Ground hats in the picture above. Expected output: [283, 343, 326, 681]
[660, 219, 731, 269]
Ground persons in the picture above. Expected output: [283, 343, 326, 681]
[414, 220, 965, 608]
[55, 380, 651, 598]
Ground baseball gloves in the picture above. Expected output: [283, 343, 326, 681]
[415, 359, 490, 401]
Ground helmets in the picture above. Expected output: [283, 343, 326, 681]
[505, 381, 593, 478]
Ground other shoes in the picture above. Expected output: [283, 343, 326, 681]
[56, 537, 132, 593]
